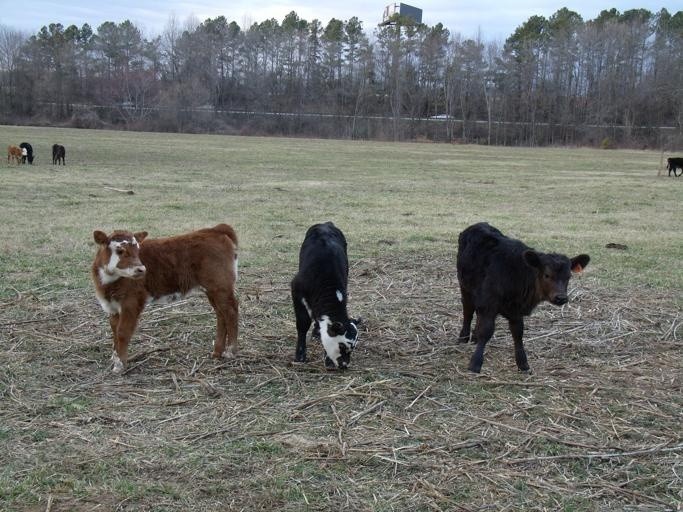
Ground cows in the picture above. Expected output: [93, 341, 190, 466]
[8, 145, 28, 168]
[89, 223, 240, 374]
[19, 142, 34, 165]
[666, 157, 683, 177]
[52, 143, 66, 165]
[289, 220, 362, 372]
[456, 221, 591, 376]
